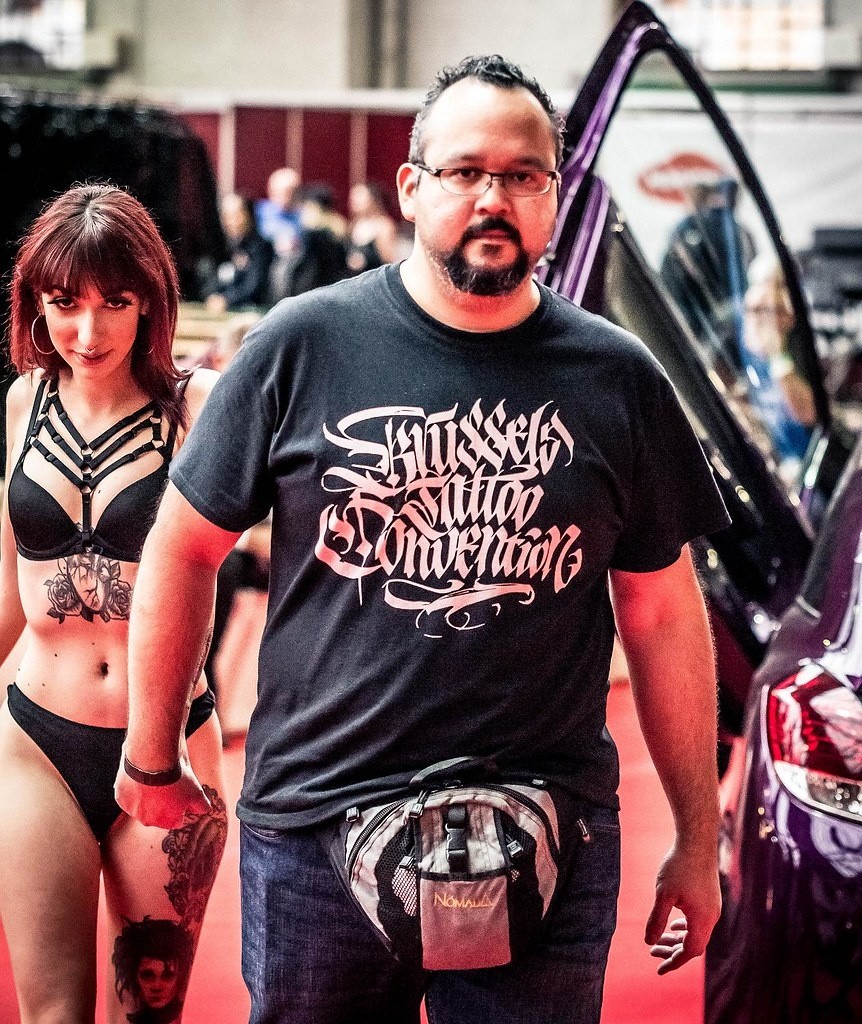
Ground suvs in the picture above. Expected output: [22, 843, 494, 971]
[2, 73, 243, 426]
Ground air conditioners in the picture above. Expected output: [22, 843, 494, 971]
[1, 0, 91, 44]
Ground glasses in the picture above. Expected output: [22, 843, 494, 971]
[412, 160, 561, 197]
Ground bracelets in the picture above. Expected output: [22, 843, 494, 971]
[122, 748, 182, 787]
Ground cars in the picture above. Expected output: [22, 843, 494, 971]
[531, 0, 862, 1024]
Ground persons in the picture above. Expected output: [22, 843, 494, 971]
[738, 253, 819, 483]
[115, 54, 736, 1024]
[221, 168, 401, 310]
[663, 174, 758, 388]
[0, 176, 230, 1023]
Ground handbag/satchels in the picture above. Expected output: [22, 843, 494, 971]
[319, 759, 604, 980]
